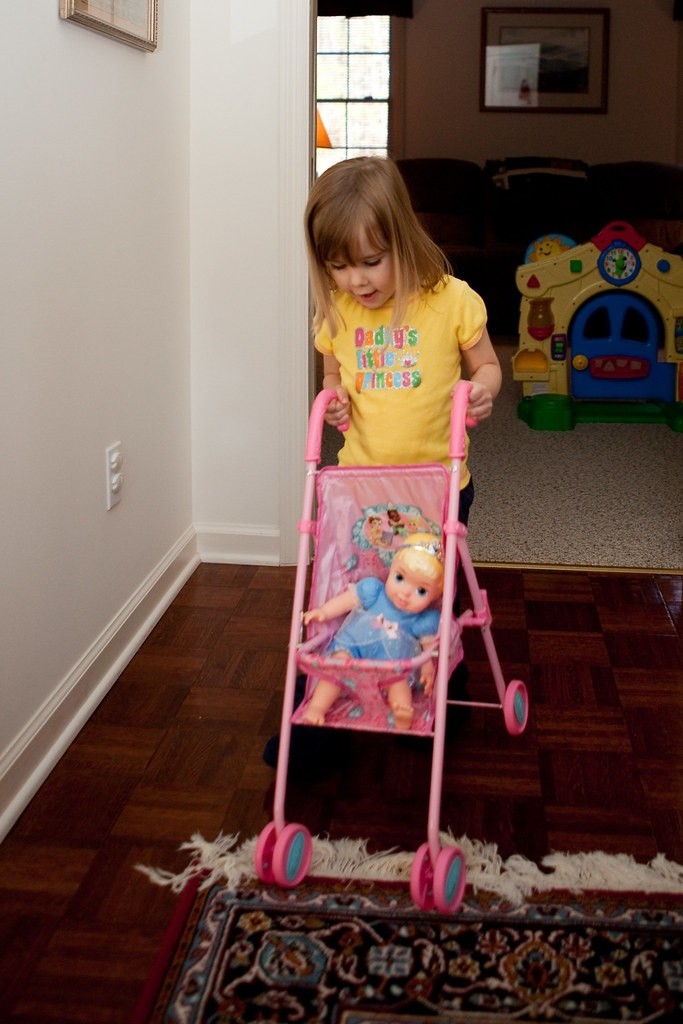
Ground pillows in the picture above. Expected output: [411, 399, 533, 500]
[414, 210, 499, 256]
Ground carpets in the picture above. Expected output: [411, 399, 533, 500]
[133, 828, 683, 1024]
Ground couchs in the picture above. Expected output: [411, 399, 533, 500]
[396, 158, 683, 333]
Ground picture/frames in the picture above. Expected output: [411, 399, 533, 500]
[58, 0, 158, 53]
[478, 6, 612, 115]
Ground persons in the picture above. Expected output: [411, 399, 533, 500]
[262, 157, 503, 771]
[298, 532, 445, 729]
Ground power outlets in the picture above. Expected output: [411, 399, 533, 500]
[105, 440, 126, 512]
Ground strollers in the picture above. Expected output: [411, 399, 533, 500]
[253, 379, 540, 918]
